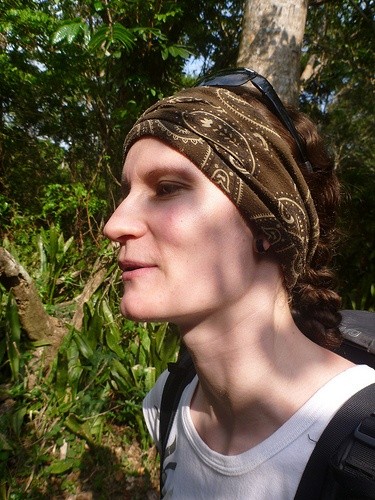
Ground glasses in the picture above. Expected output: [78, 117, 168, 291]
[199, 68, 316, 176]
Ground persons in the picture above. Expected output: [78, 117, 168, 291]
[102, 85, 374, 500]
